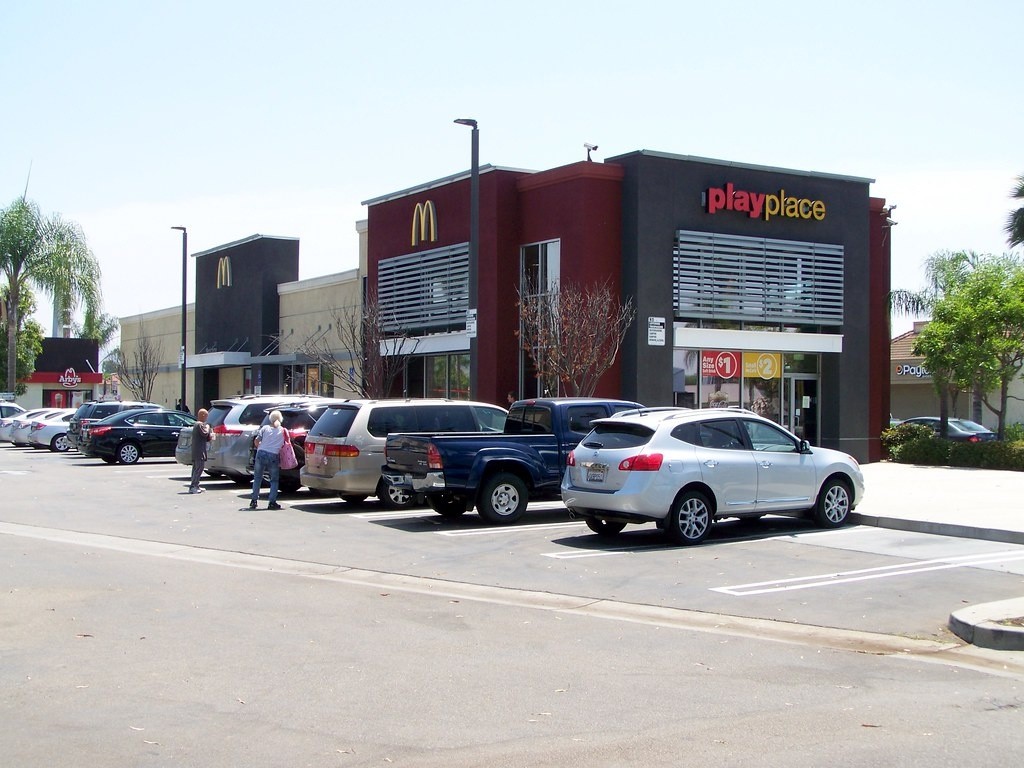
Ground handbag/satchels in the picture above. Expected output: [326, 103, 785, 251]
[280, 428, 298, 469]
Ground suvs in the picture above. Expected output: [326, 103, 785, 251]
[299, 398, 514, 510]
[66, 399, 191, 458]
[175, 394, 382, 494]
[561, 406, 865, 547]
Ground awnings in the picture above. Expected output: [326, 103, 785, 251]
[244, 353, 333, 365]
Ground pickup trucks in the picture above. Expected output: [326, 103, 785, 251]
[380, 397, 646, 525]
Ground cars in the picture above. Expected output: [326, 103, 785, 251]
[0, 398, 79, 452]
[894, 416, 999, 443]
[78, 408, 199, 465]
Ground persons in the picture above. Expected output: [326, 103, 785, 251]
[507, 391, 519, 410]
[176, 399, 191, 414]
[250, 410, 290, 509]
[188, 408, 214, 494]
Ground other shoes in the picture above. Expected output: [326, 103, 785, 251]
[250, 499, 257, 506]
[190, 486, 207, 493]
[268, 501, 280, 509]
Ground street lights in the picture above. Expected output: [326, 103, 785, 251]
[170, 226, 188, 405]
[454, 117, 479, 402]
